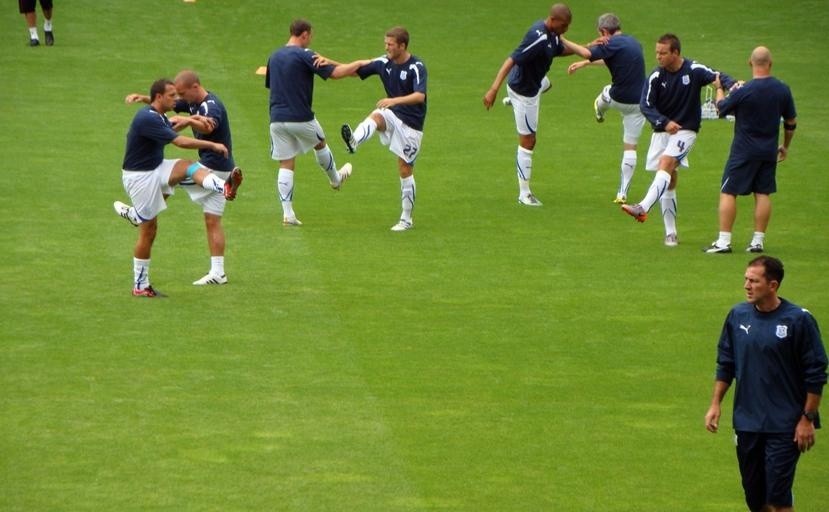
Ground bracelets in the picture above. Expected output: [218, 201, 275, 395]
[715, 87, 723, 91]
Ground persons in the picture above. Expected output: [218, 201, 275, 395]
[483, 3, 612, 207]
[112, 70, 233, 287]
[121, 77, 246, 299]
[702, 46, 796, 254]
[704, 255, 828, 512]
[558, 12, 650, 209]
[310, 26, 428, 233]
[16, 1, 56, 48]
[620, 32, 746, 247]
[261, 16, 354, 226]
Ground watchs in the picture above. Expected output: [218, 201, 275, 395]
[802, 410, 815, 421]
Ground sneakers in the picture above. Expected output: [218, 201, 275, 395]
[702, 240, 731, 253]
[331, 162, 352, 189]
[664, 235, 678, 246]
[612, 198, 626, 203]
[622, 204, 647, 222]
[193, 273, 227, 287]
[519, 191, 542, 207]
[28, 30, 54, 46]
[341, 123, 358, 153]
[503, 97, 513, 104]
[131, 285, 166, 298]
[390, 219, 414, 232]
[222, 167, 243, 200]
[113, 200, 141, 228]
[746, 243, 763, 252]
[593, 100, 603, 122]
[282, 218, 303, 226]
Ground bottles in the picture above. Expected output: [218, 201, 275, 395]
[704, 99, 715, 118]
